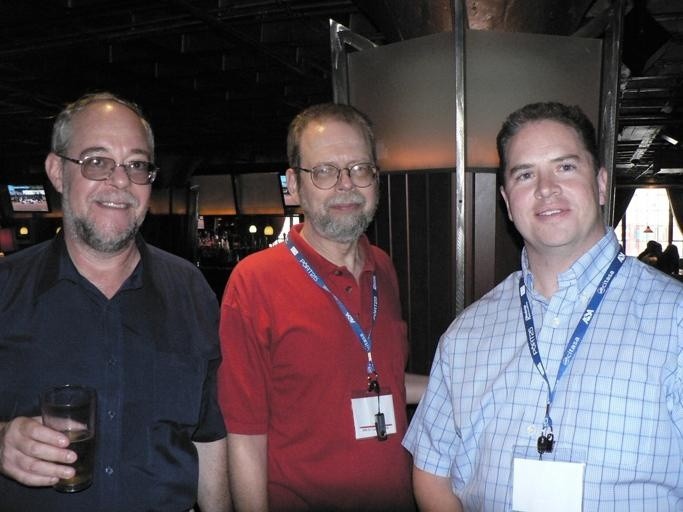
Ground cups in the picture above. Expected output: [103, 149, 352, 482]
[39, 384, 97, 493]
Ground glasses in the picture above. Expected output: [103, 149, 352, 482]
[53, 151, 158, 186]
[291, 160, 382, 192]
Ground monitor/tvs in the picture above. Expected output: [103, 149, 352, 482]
[278, 173, 300, 207]
[6, 184, 51, 213]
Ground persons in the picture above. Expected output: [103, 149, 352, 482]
[653, 244, 679, 278]
[639, 242, 662, 265]
[637, 240, 657, 260]
[0, 92, 234, 512]
[396, 98, 682, 512]
[215, 101, 420, 512]
